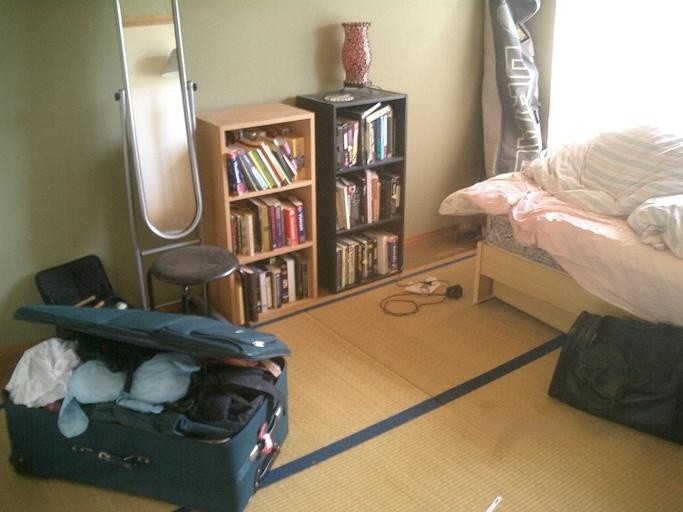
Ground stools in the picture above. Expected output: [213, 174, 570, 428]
[148, 246, 249, 327]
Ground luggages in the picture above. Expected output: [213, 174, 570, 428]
[3, 303, 290, 512]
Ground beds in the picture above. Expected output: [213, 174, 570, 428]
[439, 130, 683, 336]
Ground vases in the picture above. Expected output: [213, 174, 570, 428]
[343, 21, 371, 87]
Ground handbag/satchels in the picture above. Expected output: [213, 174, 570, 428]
[548, 310, 683, 445]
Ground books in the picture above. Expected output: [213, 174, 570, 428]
[230, 194, 307, 256]
[235, 252, 310, 326]
[335, 232, 400, 290]
[225, 130, 308, 197]
[336, 101, 397, 166]
[335, 170, 402, 230]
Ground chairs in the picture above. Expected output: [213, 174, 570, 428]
[35, 256, 130, 313]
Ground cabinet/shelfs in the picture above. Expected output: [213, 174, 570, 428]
[196, 102, 318, 326]
[296, 86, 408, 292]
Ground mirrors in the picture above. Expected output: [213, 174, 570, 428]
[114, 1, 204, 240]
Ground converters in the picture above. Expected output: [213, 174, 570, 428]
[447, 284, 463, 300]
